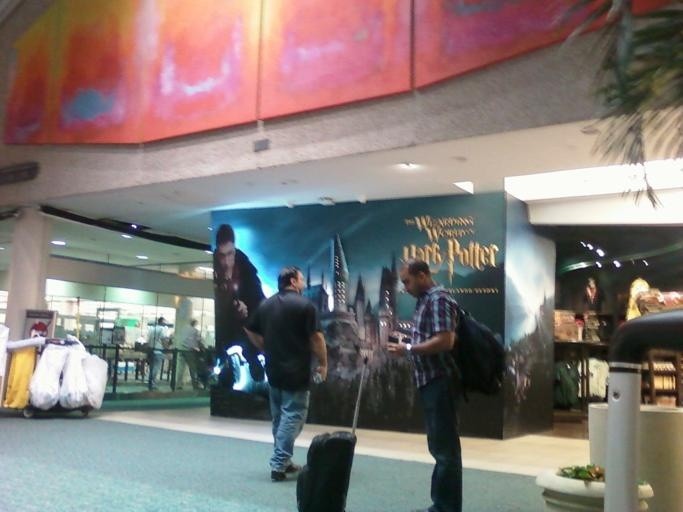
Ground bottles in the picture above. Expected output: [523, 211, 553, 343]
[313, 372, 322, 386]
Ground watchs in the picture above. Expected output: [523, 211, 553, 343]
[405, 342, 412, 356]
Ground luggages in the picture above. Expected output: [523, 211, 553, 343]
[296, 356, 368, 512]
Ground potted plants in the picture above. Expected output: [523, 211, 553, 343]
[535, 464, 654, 512]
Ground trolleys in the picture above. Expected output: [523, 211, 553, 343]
[0, 352, 94, 419]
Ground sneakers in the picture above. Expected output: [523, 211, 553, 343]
[287, 463, 301, 472]
[271, 471, 286, 480]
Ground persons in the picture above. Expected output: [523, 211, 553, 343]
[384, 259, 465, 512]
[239, 266, 328, 482]
[144, 317, 169, 391]
[211, 224, 266, 390]
[181, 319, 204, 390]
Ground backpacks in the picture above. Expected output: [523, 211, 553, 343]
[455, 310, 505, 394]
